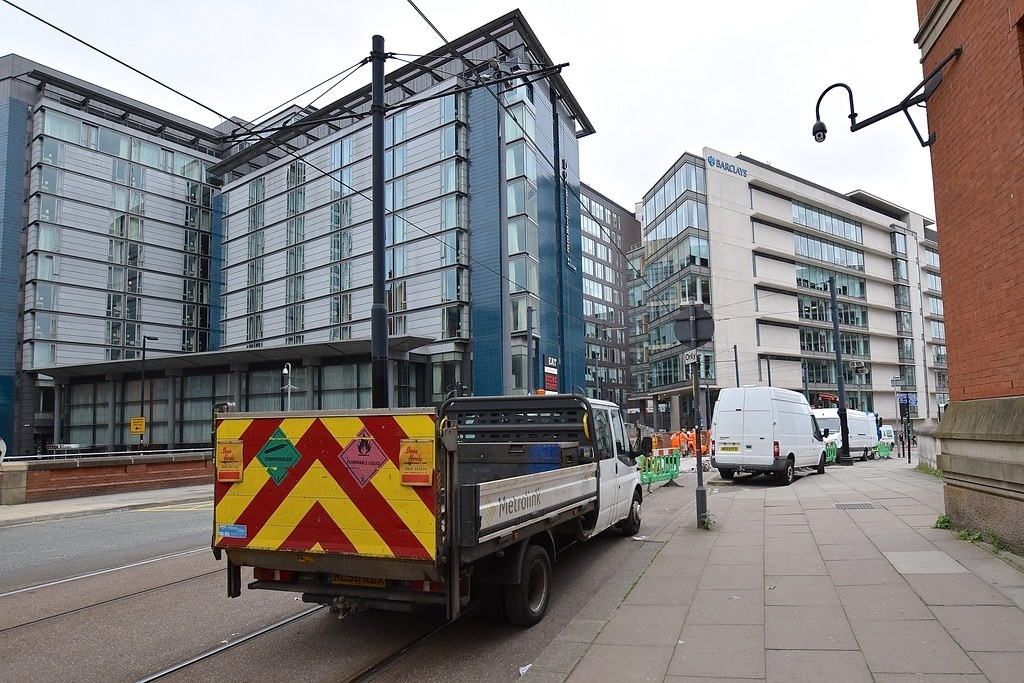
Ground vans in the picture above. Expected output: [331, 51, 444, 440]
[810, 407, 879, 461]
[879, 424, 895, 451]
[708, 384, 830, 486]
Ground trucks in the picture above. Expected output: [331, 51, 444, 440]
[205, 390, 654, 628]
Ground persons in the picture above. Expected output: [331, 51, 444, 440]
[651, 425, 712, 456]
[912, 433, 917, 447]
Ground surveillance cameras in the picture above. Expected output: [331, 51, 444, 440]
[282, 368, 288, 376]
[812, 121, 827, 143]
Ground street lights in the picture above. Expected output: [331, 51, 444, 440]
[893, 375, 901, 458]
[137, 334, 159, 451]
[283, 362, 291, 411]
